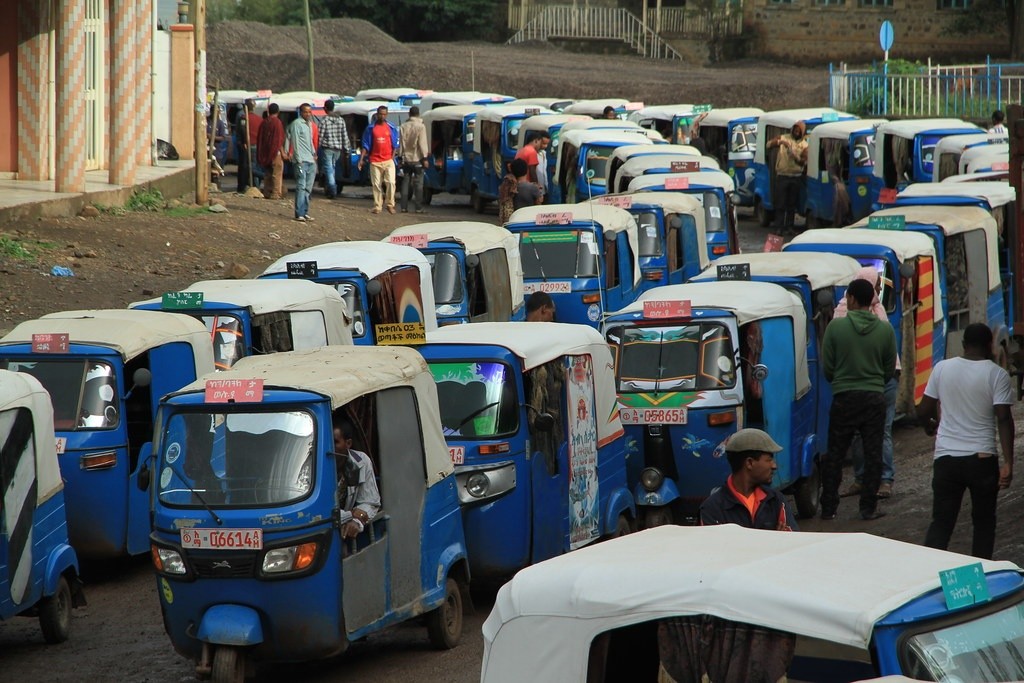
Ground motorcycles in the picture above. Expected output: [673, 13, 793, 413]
[203, 84, 1020, 467]
[587, 282, 818, 537]
[123, 274, 356, 374]
[258, 241, 438, 355]
[0, 369, 91, 643]
[138, 344, 472, 683]
[403, 319, 638, 596]
[480, 523, 1023, 682]
[0, 310, 220, 577]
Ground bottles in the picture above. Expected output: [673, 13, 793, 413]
[788, 147, 793, 160]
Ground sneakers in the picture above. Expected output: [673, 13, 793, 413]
[304, 215, 314, 220]
[294, 216, 306, 221]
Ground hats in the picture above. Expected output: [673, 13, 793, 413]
[725, 428, 783, 452]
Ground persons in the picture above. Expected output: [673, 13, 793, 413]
[256, 103, 288, 198]
[821, 279, 897, 519]
[988, 110, 1008, 144]
[920, 323, 1015, 560]
[358, 107, 403, 215]
[500, 159, 544, 223]
[516, 132, 551, 195]
[398, 106, 428, 212]
[206, 104, 225, 184]
[243, 98, 264, 187]
[834, 274, 902, 497]
[767, 121, 808, 236]
[661, 121, 674, 143]
[318, 100, 350, 199]
[603, 106, 615, 119]
[291, 103, 317, 222]
[525, 291, 556, 322]
[700, 430, 800, 531]
[330, 419, 381, 539]
[289, 120, 319, 199]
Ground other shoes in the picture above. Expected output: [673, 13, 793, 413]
[401, 208, 408, 212]
[822, 512, 836, 519]
[387, 206, 396, 214]
[864, 505, 886, 519]
[374, 209, 380, 213]
[415, 209, 427, 212]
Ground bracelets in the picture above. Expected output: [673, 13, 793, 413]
[354, 512, 366, 530]
